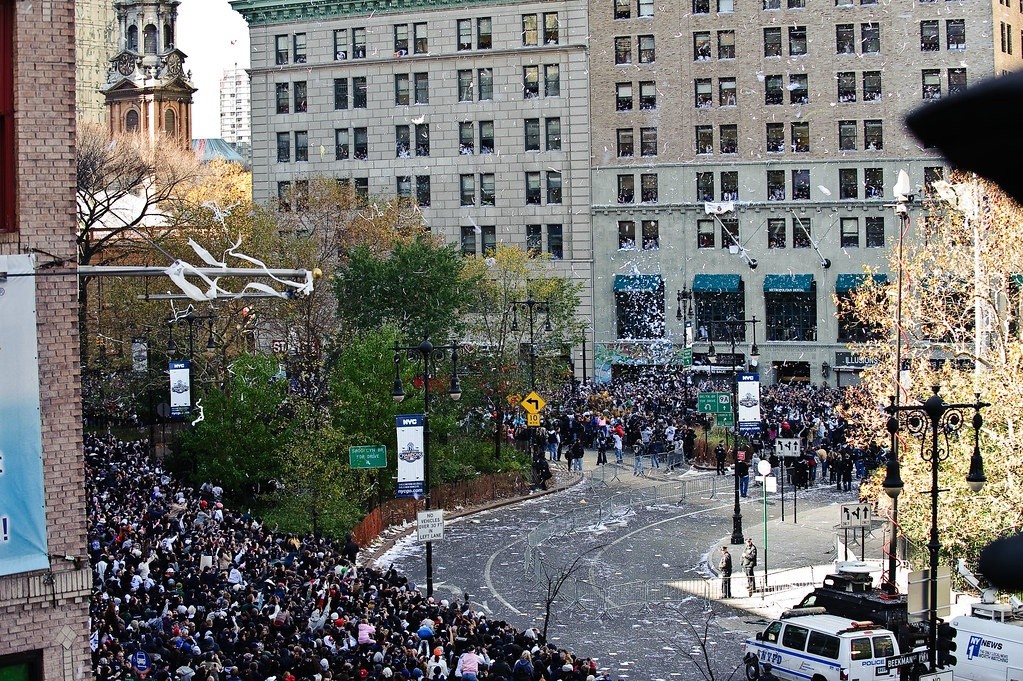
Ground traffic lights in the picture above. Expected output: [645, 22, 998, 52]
[941, 625, 957, 666]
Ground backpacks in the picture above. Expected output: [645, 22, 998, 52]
[576, 448, 584, 458]
[564, 451, 570, 460]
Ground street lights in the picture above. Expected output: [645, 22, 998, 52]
[678, 285, 698, 419]
[890, 169, 914, 461]
[511, 293, 556, 391]
[163, 309, 220, 370]
[884, 380, 992, 669]
[387, 334, 465, 601]
[705, 313, 762, 545]
[756, 460, 772, 591]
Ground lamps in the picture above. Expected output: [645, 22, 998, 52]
[821, 361, 830, 378]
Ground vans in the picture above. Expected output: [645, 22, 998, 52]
[950, 590, 1023, 680]
[795, 568, 931, 681]
[742, 608, 903, 681]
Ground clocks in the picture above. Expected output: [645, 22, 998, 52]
[167, 52, 182, 75]
[118, 54, 135, 76]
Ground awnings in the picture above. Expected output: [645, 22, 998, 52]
[612, 274, 662, 293]
[692, 274, 742, 291]
[1009, 271, 1023, 290]
[921, 274, 969, 293]
[763, 274, 814, 293]
[836, 274, 888, 294]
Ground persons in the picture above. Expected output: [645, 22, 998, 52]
[82, 422, 606, 681]
[741, 538, 758, 594]
[281, 35, 960, 342]
[758, 663, 780, 681]
[454, 377, 886, 498]
[719, 547, 732, 598]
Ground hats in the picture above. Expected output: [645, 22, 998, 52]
[674, 436, 682, 441]
[745, 538, 753, 542]
[575, 439, 580, 441]
[719, 440, 725, 444]
[770, 452, 774, 455]
[720, 546, 728, 551]
[636, 439, 642, 444]
